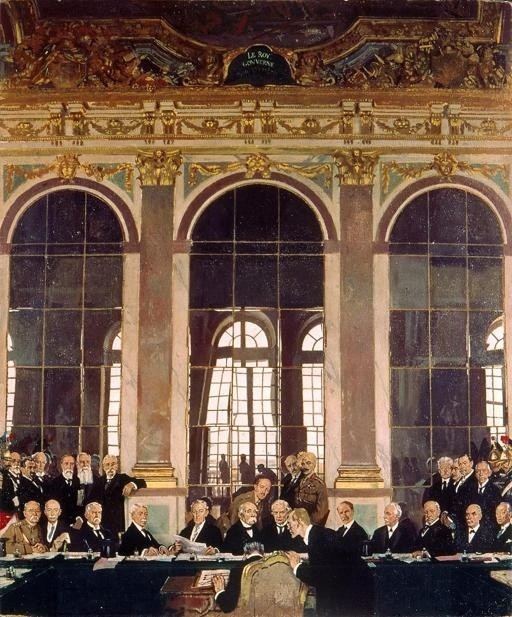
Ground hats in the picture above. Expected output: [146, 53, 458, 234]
[486, 448, 512, 462]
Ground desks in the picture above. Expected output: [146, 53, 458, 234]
[0, 550, 512, 617]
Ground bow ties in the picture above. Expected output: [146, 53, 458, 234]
[386, 527, 395, 533]
[243, 525, 254, 531]
[468, 529, 476, 534]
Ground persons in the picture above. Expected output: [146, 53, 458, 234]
[284, 506, 374, 615]
[207, 541, 267, 614]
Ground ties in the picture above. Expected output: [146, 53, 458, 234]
[191, 524, 203, 541]
[141, 527, 151, 543]
[93, 528, 103, 539]
[48, 525, 56, 540]
[275, 523, 285, 534]
[339, 526, 349, 537]
[439, 475, 493, 495]
[9, 474, 79, 491]
[495, 527, 504, 539]
[286, 477, 297, 494]
[420, 524, 430, 535]
